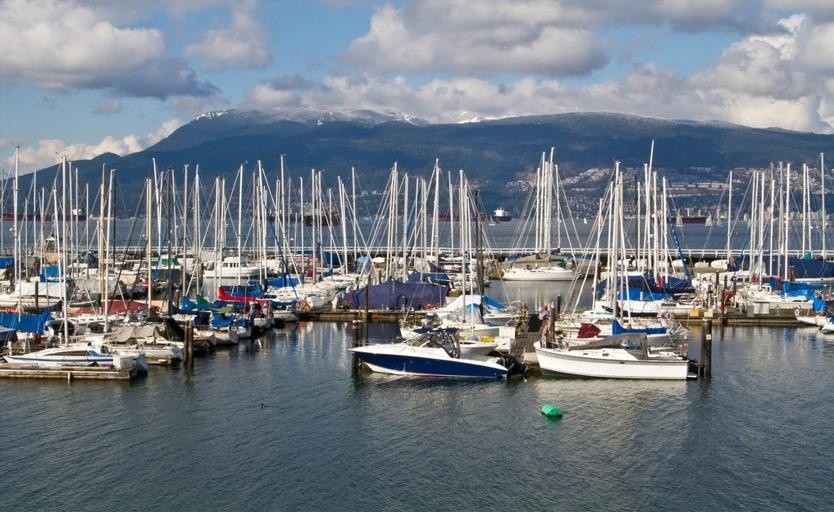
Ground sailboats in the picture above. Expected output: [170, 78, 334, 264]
[500, 139, 833, 382]
[1, 146, 526, 383]
